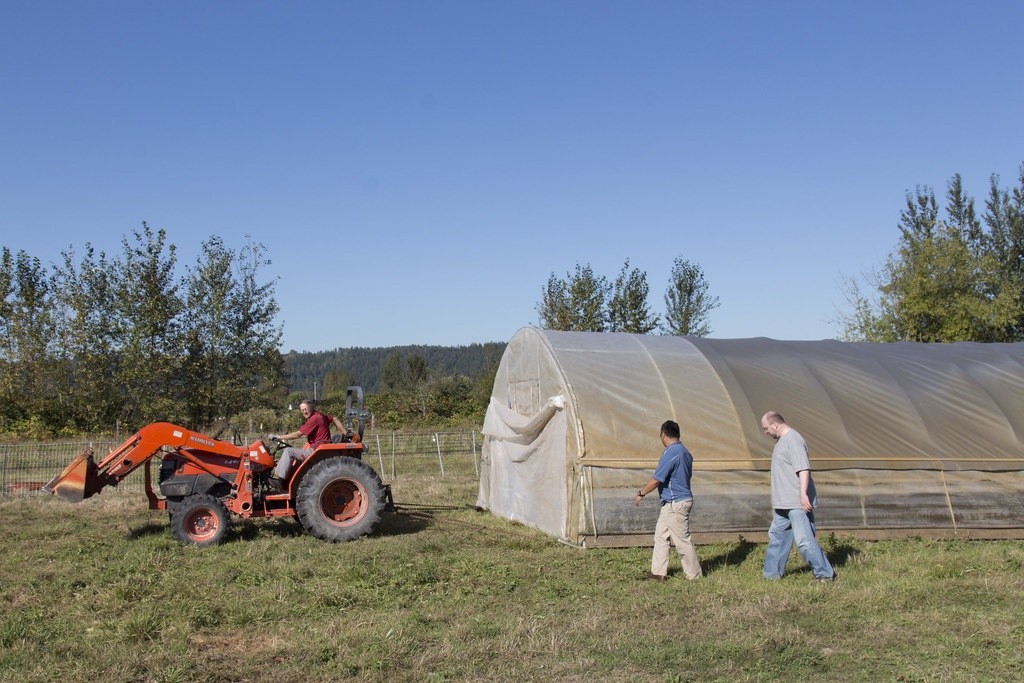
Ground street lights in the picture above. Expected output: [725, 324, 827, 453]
[313, 382, 318, 400]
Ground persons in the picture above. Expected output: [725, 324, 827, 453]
[761, 410, 837, 582]
[268, 399, 348, 490]
[633, 419, 706, 581]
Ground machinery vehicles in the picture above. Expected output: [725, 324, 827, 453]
[41, 386, 387, 546]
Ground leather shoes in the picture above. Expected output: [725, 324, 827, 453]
[647, 571, 667, 582]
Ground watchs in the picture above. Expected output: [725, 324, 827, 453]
[637, 491, 645, 497]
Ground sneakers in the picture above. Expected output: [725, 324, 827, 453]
[268, 478, 283, 493]
